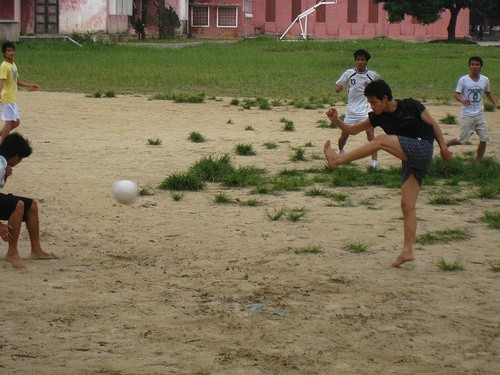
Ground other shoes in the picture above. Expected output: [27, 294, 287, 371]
[338, 149, 345, 154]
[372, 160, 377, 166]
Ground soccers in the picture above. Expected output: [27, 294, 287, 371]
[112, 180, 139, 205]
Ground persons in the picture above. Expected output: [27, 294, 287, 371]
[441, 56, 500, 169]
[0, 39, 40, 150]
[0, 132, 60, 270]
[333, 49, 390, 174]
[322, 79, 455, 269]
